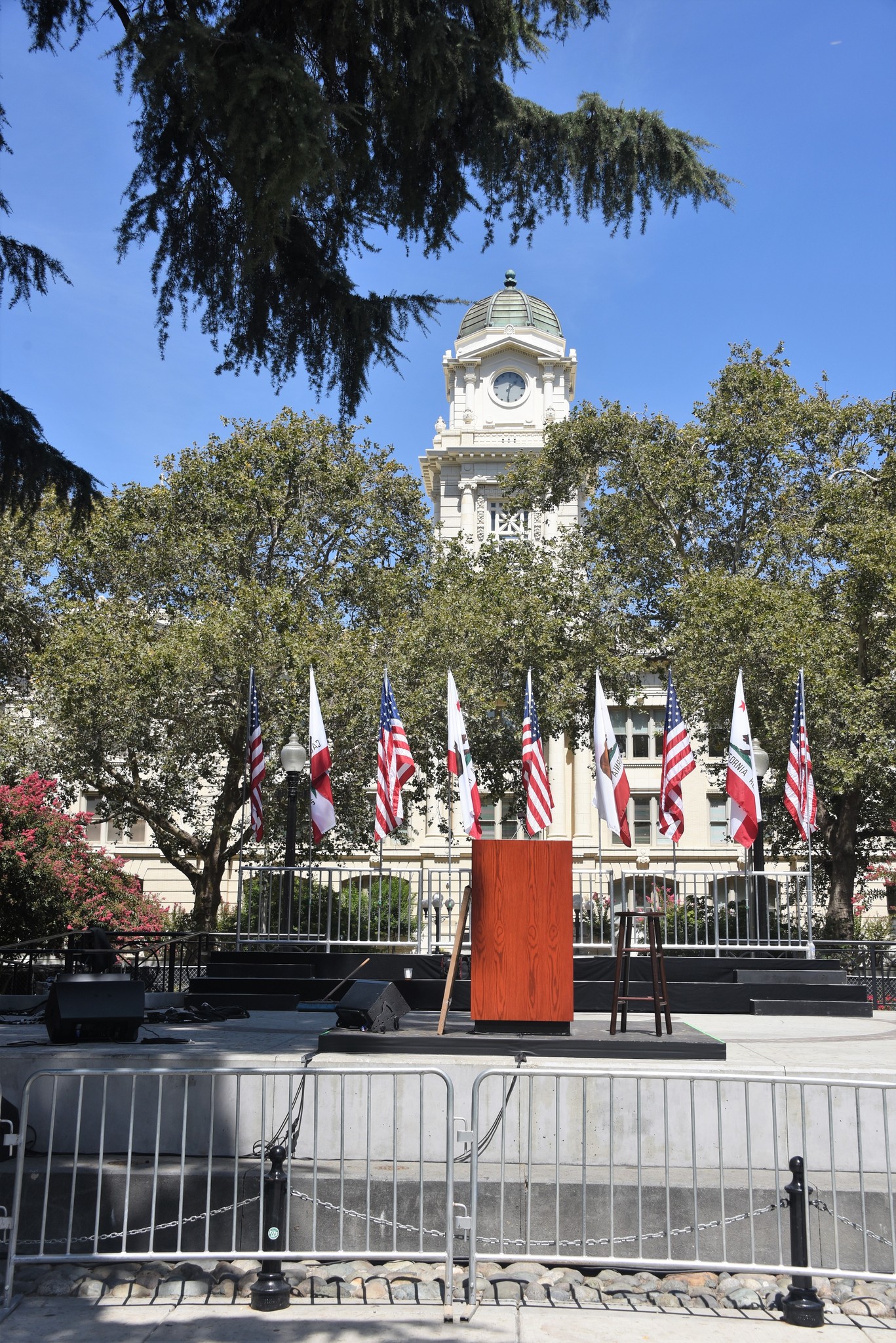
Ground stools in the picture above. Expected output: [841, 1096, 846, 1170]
[610, 912, 673, 1036]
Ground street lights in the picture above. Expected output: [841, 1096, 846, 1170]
[751, 738, 769, 950]
[282, 727, 307, 942]
[421, 891, 455, 956]
[572, 894, 595, 956]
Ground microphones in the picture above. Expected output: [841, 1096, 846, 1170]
[511, 812, 532, 840]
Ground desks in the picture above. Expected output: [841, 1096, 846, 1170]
[471, 840, 573, 1036]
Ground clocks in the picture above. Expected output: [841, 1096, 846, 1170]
[493, 372, 526, 403]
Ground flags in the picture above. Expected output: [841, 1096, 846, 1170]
[375, 676, 416, 842]
[593, 671, 631, 848]
[447, 669, 482, 840]
[659, 669, 696, 841]
[522, 670, 555, 836]
[784, 672, 819, 841]
[725, 669, 763, 848]
[308, 669, 336, 842]
[248, 677, 268, 843]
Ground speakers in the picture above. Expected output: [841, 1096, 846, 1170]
[335, 979, 411, 1030]
[44, 974, 144, 1043]
